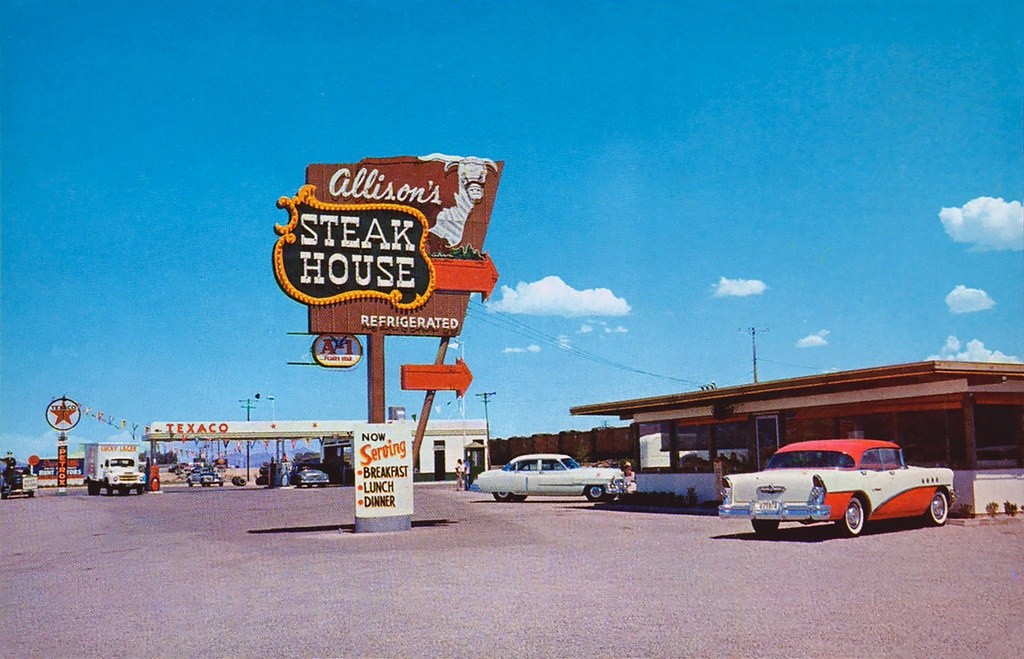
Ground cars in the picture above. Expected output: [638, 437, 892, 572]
[469, 454, 624, 501]
[188, 470, 224, 487]
[718, 439, 954, 536]
[294, 469, 331, 488]
[1, 467, 34, 499]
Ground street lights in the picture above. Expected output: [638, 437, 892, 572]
[265, 394, 276, 420]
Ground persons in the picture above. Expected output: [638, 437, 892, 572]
[455, 458, 470, 491]
[0, 451, 16, 485]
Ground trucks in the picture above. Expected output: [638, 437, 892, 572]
[82, 442, 147, 496]
